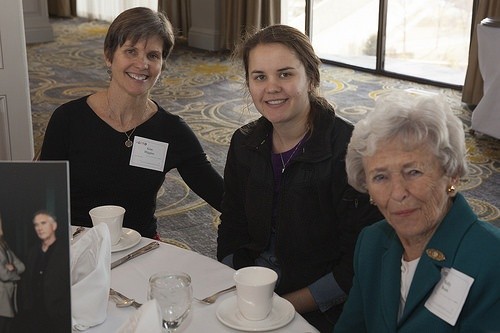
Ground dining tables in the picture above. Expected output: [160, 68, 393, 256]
[72, 226, 321, 333]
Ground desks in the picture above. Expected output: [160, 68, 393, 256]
[470, 18, 500, 140]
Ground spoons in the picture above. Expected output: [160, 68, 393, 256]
[109, 295, 135, 308]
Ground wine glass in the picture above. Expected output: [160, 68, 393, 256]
[148, 271, 192, 333]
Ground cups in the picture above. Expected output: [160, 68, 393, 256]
[232, 266, 278, 321]
[88, 205, 126, 246]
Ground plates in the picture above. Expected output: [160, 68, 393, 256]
[111, 228, 141, 253]
[216, 295, 295, 332]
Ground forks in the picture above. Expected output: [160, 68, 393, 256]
[193, 285, 237, 304]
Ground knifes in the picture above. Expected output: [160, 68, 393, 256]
[111, 242, 160, 270]
